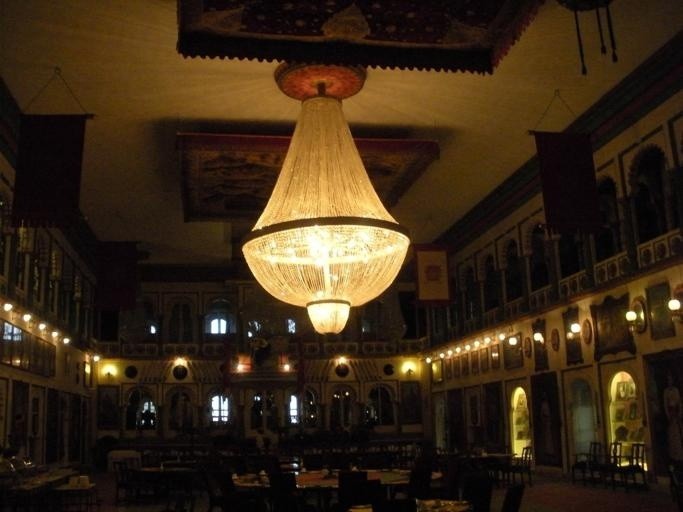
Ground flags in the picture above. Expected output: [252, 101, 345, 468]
[413, 243, 452, 310]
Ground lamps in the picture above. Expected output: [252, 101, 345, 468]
[239, 60, 412, 338]
[567, 286, 683, 344]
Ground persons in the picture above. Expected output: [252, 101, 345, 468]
[661, 372, 683, 462]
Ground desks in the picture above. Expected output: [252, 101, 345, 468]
[54, 484, 98, 511]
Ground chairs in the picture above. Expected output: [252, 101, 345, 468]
[112, 441, 650, 512]
